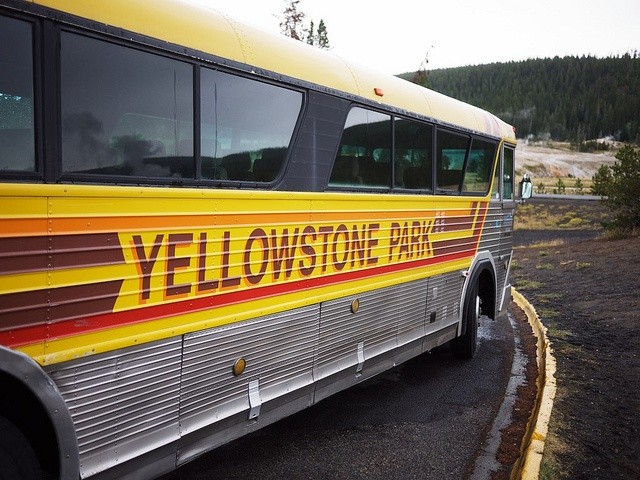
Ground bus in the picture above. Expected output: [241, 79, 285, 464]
[0, 0, 532, 480]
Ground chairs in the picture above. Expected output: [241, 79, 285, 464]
[357, 156, 379, 185]
[218, 147, 252, 181]
[333, 156, 363, 186]
[175, 138, 222, 181]
[123, 139, 164, 180]
